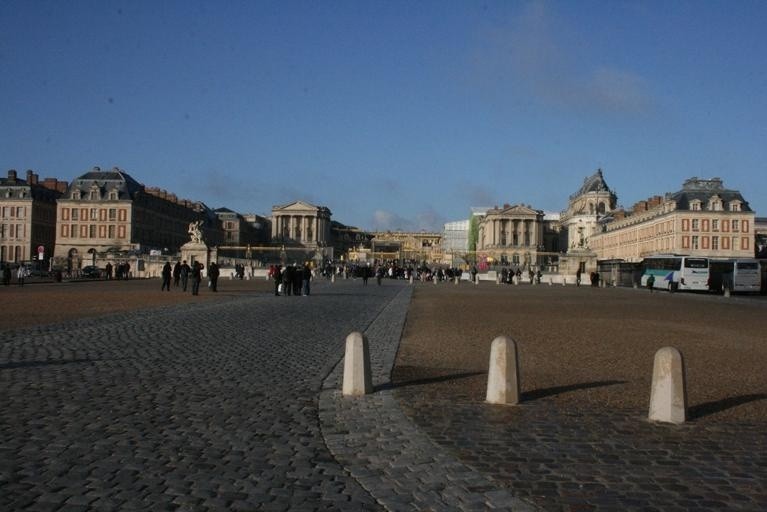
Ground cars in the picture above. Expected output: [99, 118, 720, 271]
[81, 265, 106, 278]
[759, 259, 767, 294]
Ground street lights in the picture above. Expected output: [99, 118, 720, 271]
[90, 247, 96, 279]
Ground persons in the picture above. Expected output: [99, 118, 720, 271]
[590, 271, 600, 288]
[105, 260, 130, 280]
[48, 268, 101, 281]
[648, 274, 655, 293]
[313, 261, 479, 284]
[492, 259, 542, 286]
[161, 261, 255, 296]
[268, 261, 312, 296]
[4, 261, 32, 287]
[188, 217, 204, 241]
[576, 268, 582, 288]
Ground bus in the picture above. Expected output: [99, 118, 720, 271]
[634, 253, 711, 293]
[707, 258, 762, 295]
